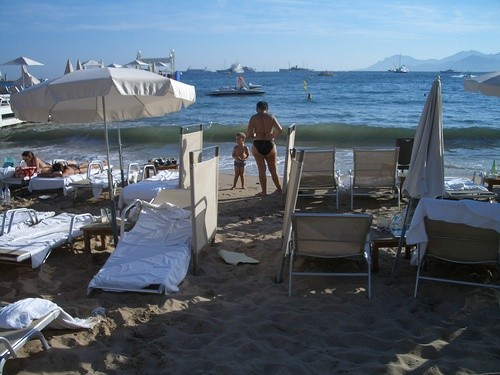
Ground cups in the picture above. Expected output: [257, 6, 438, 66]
[101, 208, 109, 223]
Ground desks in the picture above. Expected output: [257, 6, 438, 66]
[79, 220, 135, 254]
[483, 176, 500, 191]
[370, 228, 411, 272]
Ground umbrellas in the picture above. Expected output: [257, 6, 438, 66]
[64, 59, 167, 75]
[10, 67, 196, 246]
[388, 75, 445, 280]
[3, 56, 44, 85]
[464, 73, 500, 96]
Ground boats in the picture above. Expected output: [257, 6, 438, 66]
[440, 69, 454, 73]
[185, 67, 207, 73]
[208, 89, 266, 96]
[279, 65, 310, 71]
[232, 64, 245, 74]
[388, 54, 408, 74]
[449, 73, 477, 78]
[216, 63, 255, 74]
[0, 95, 27, 127]
[221, 83, 263, 90]
[318, 70, 335, 77]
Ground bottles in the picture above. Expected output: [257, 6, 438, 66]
[0, 185, 4, 204]
[4, 184, 10, 205]
[489, 160, 497, 174]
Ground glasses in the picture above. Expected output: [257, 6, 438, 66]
[22, 159, 30, 161]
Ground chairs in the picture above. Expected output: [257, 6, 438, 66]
[288, 148, 500, 298]
[0, 159, 195, 375]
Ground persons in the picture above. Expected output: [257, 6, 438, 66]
[231, 132, 249, 190]
[52, 161, 108, 174]
[22, 151, 77, 174]
[247, 101, 283, 197]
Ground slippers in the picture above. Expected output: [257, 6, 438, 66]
[276, 190, 282, 193]
[256, 193, 265, 196]
